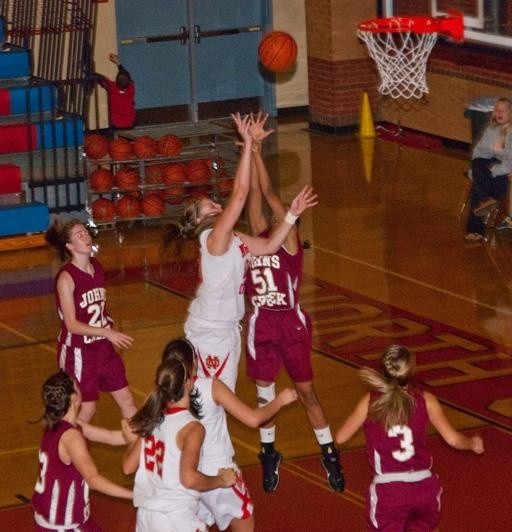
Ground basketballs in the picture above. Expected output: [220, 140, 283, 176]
[84, 135, 211, 222]
[259, 31, 296, 71]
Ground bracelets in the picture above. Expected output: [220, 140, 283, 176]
[282, 210, 300, 226]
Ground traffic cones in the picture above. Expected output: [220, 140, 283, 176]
[357, 92, 379, 139]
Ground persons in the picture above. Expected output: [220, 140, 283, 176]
[335, 341, 486, 531]
[40, 215, 139, 427]
[153, 334, 302, 531]
[229, 109, 346, 496]
[157, 111, 320, 395]
[20, 366, 136, 530]
[116, 357, 239, 531]
[93, 52, 139, 142]
[460, 97, 511, 243]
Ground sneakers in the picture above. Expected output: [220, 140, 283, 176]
[466, 232, 481, 240]
[321, 450, 345, 492]
[497, 215, 512, 229]
[473, 198, 497, 216]
[258, 451, 283, 494]
